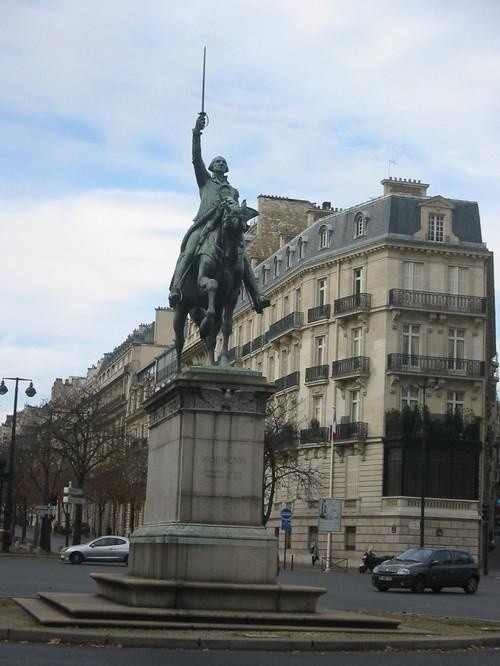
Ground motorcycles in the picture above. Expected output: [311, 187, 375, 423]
[358, 549, 396, 575]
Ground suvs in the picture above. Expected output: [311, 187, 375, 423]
[371, 544, 481, 596]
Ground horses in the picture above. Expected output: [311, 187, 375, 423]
[174, 197, 260, 374]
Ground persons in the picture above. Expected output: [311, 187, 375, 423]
[310, 544, 318, 565]
[168, 116, 271, 313]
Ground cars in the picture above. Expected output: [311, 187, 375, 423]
[57, 534, 131, 566]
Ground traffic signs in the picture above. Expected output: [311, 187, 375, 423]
[35, 505, 54, 510]
[63, 487, 83, 495]
[63, 496, 85, 504]
[38, 510, 55, 515]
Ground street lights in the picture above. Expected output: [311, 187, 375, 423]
[0, 374, 38, 557]
[400, 371, 447, 549]
